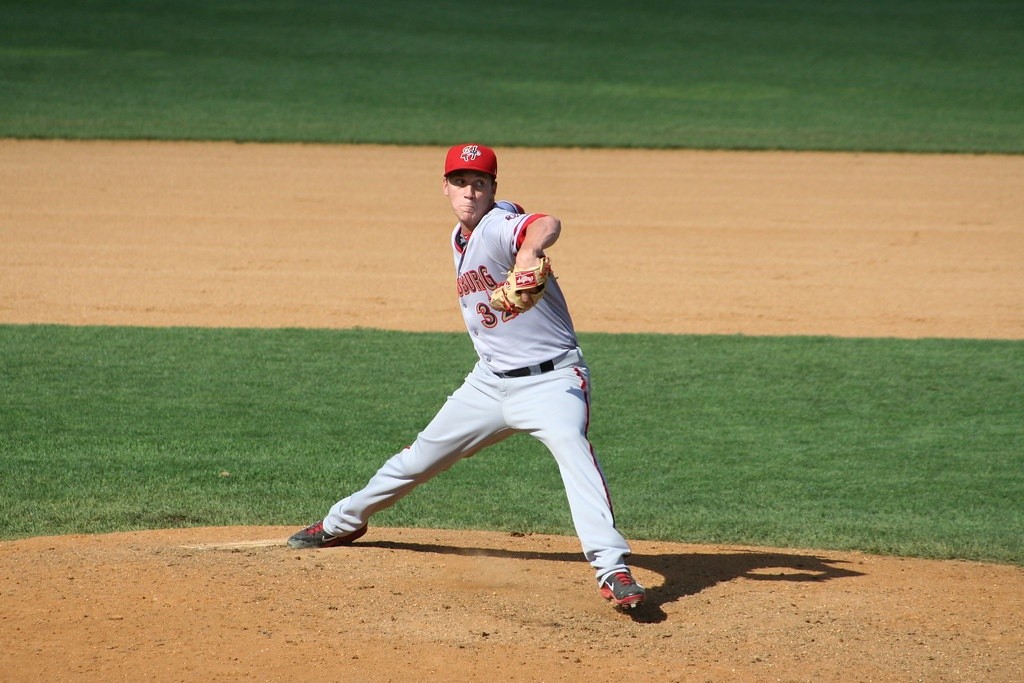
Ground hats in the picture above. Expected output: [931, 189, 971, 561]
[444, 143, 497, 178]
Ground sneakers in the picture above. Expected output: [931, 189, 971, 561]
[601, 572, 646, 609]
[287, 519, 368, 550]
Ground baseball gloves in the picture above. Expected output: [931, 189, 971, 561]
[489, 255, 553, 315]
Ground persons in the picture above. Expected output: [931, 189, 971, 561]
[287, 144, 645, 606]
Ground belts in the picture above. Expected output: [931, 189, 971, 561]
[493, 359, 554, 379]
